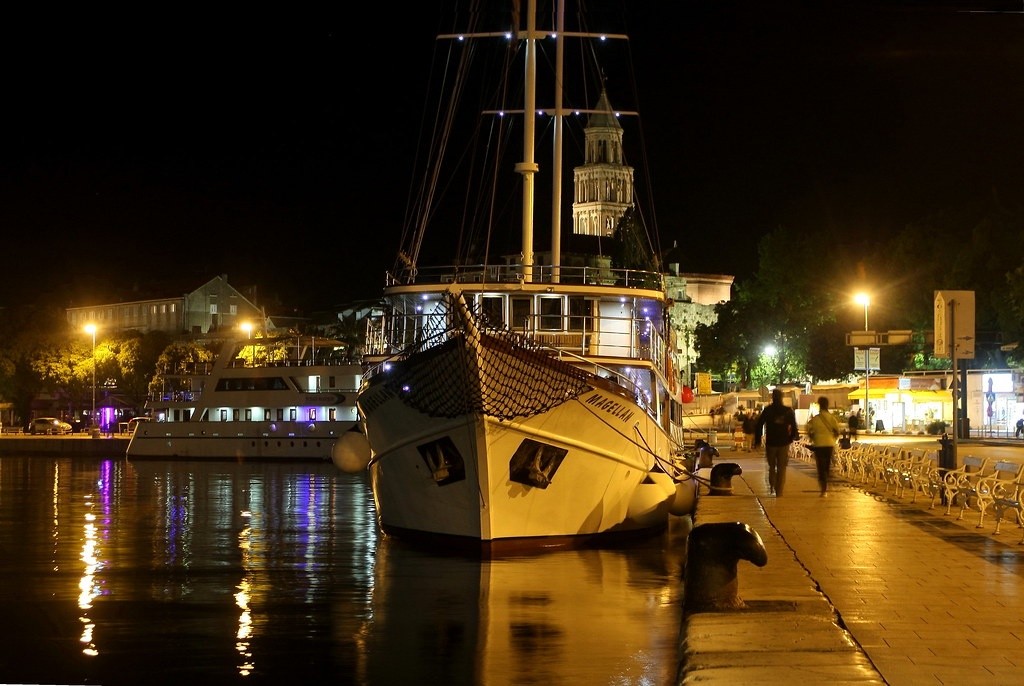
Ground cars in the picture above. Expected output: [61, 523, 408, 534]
[28, 418, 73, 435]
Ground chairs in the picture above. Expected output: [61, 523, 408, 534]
[787, 434, 1024, 546]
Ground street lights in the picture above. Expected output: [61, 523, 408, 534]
[242, 324, 255, 368]
[86, 326, 95, 425]
[764, 345, 784, 394]
[856, 294, 869, 431]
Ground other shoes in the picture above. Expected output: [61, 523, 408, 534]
[776, 494, 783, 498]
[819, 491, 827, 499]
[770, 486, 775, 494]
[741, 448, 746, 451]
[748, 450, 753, 453]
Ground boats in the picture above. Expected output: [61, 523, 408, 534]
[124, 334, 364, 463]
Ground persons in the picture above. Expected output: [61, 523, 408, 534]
[849, 411, 857, 441]
[806, 396, 839, 495]
[741, 409, 761, 451]
[859, 408, 862, 418]
[754, 389, 799, 496]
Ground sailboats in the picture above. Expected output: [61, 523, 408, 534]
[354, 1, 683, 551]
